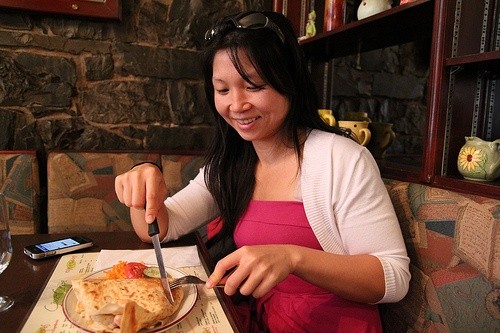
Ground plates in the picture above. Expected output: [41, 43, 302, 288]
[61, 263, 199, 333]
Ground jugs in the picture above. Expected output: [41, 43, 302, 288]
[458, 136, 500, 181]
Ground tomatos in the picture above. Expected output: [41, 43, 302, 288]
[124, 262, 148, 279]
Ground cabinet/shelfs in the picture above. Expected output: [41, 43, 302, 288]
[273, 0, 500, 202]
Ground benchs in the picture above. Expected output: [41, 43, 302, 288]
[0, 149, 500, 333]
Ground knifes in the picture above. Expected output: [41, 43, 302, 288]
[143, 203, 175, 306]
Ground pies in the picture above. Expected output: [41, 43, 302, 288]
[71, 277, 184, 333]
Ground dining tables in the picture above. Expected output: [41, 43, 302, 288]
[0, 231, 247, 333]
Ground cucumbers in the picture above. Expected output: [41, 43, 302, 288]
[143, 267, 168, 278]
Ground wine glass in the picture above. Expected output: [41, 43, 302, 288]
[0, 194, 15, 311]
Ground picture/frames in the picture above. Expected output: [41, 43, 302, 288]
[0, 0, 130, 25]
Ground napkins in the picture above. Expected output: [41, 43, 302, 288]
[93, 245, 201, 272]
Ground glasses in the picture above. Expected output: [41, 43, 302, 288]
[204, 12, 286, 45]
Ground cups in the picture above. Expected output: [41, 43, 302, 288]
[318, 109, 335, 126]
[338, 121, 372, 146]
[367, 122, 395, 161]
[347, 112, 371, 122]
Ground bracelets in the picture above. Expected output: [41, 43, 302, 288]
[131, 162, 162, 172]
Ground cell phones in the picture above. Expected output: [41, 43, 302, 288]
[23, 235, 93, 260]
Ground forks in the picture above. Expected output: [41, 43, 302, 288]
[170, 275, 225, 290]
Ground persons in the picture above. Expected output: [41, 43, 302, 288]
[115, 11, 411, 333]
